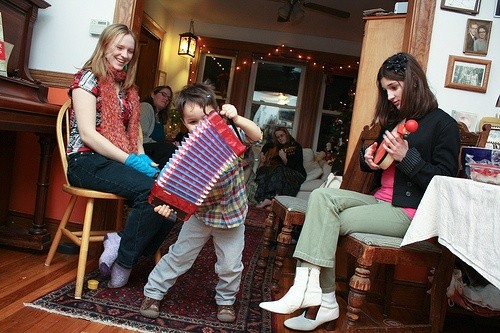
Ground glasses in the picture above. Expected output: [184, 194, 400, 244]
[159, 91, 172, 101]
[276, 134, 286, 139]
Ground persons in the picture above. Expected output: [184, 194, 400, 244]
[255, 127, 306, 210]
[260, 52, 462, 331]
[466, 23, 488, 53]
[66, 23, 179, 290]
[140, 86, 177, 168]
[139, 80, 262, 322]
[457, 69, 479, 86]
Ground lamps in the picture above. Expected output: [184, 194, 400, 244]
[278, 4, 306, 25]
[178, 19, 198, 58]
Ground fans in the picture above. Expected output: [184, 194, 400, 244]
[277, 0, 351, 23]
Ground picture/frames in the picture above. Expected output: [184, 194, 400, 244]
[463, 18, 494, 56]
[440, 0, 481, 15]
[444, 55, 493, 94]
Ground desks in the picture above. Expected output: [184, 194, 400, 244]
[400, 174, 500, 333]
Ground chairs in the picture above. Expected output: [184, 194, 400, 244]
[255, 115, 491, 333]
[43, 99, 160, 301]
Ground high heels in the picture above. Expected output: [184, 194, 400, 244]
[284, 290, 340, 331]
[259, 267, 323, 321]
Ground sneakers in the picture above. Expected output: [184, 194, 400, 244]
[139, 296, 161, 318]
[217, 305, 236, 322]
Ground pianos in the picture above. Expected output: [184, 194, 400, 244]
[0, 0, 68, 249]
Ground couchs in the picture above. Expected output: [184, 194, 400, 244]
[250, 147, 332, 197]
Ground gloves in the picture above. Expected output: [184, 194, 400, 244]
[125, 153, 160, 178]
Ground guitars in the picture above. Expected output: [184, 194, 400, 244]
[372, 119, 420, 169]
[257, 146, 297, 168]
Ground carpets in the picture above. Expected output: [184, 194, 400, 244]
[23, 204, 278, 333]
